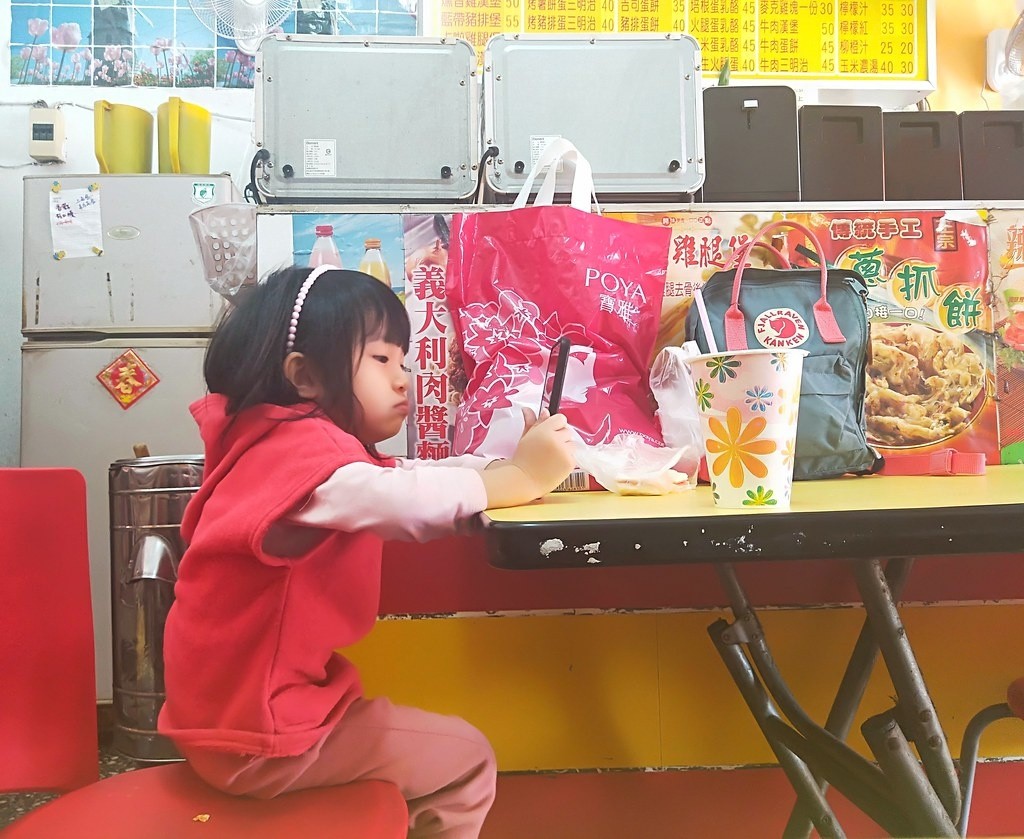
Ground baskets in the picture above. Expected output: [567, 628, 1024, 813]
[188, 203, 256, 308]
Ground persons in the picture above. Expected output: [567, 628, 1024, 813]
[158, 268, 578, 839]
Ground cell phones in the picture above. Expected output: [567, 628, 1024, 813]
[539, 337, 571, 420]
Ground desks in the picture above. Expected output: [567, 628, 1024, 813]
[476, 464, 1024, 837]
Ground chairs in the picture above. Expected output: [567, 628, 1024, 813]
[0, 468, 410, 839]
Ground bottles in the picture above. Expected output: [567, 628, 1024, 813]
[308, 224, 392, 289]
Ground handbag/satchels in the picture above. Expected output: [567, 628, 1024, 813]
[683, 219, 886, 481]
[446, 134, 676, 492]
[647, 339, 706, 460]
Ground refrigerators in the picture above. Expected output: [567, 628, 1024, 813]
[19, 173, 256, 707]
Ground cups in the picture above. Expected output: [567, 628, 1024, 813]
[157, 96, 210, 175]
[93, 99, 154, 174]
[687, 349, 808, 508]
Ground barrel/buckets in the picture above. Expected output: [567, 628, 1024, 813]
[107, 454, 205, 763]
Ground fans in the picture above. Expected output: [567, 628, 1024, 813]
[187, 0, 298, 55]
[986, 8, 1024, 92]
